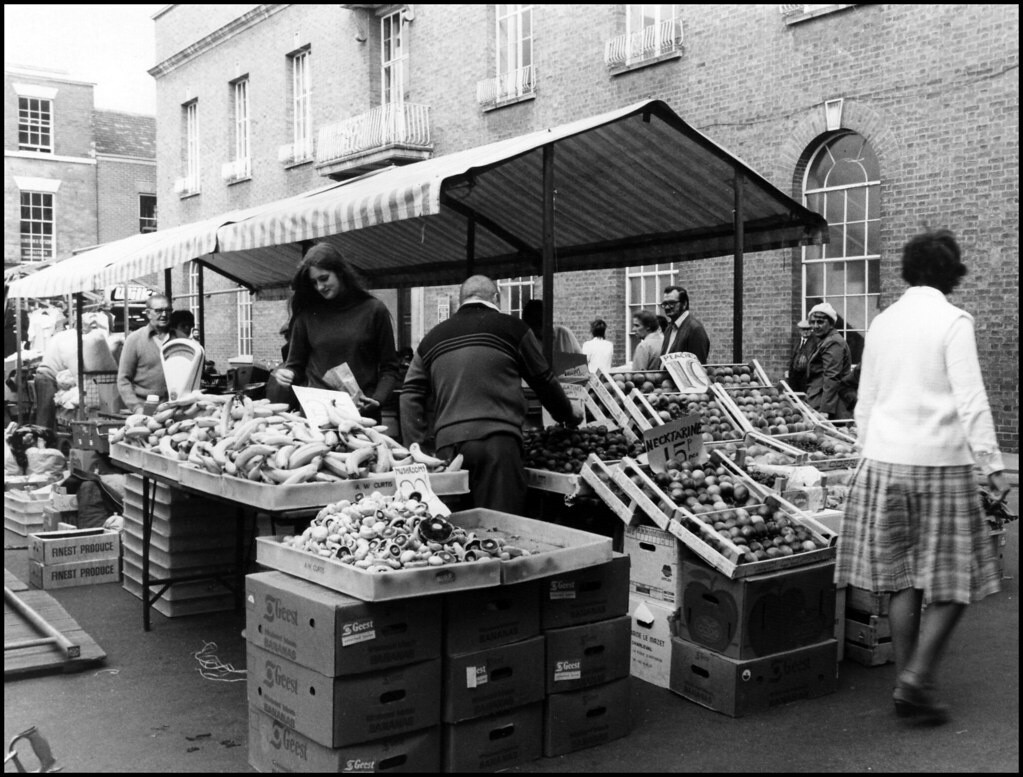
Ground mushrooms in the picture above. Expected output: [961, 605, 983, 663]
[278, 491, 534, 570]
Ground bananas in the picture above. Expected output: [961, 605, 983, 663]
[107, 390, 463, 487]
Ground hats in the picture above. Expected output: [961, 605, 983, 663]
[808, 303, 838, 325]
[797, 320, 812, 328]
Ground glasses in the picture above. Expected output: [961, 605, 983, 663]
[659, 299, 680, 308]
[150, 306, 173, 313]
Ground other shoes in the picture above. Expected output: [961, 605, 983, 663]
[891, 676, 949, 726]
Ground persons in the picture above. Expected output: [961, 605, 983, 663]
[582, 319, 613, 373]
[172, 310, 205, 378]
[658, 286, 710, 371]
[789, 303, 852, 427]
[399, 275, 584, 515]
[33, 329, 77, 434]
[116, 295, 178, 415]
[833, 230, 1011, 721]
[279, 323, 290, 363]
[657, 316, 668, 341]
[270, 242, 398, 427]
[633, 309, 662, 371]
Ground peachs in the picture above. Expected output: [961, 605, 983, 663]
[628, 457, 818, 564]
[589, 362, 860, 465]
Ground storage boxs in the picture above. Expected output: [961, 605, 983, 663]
[4, 419, 893, 775]
[553, 352, 588, 383]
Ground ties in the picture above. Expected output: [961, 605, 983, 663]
[795, 340, 805, 367]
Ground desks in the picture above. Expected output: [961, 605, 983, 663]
[110, 459, 461, 631]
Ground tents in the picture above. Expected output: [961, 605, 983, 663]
[6, 98, 828, 427]
